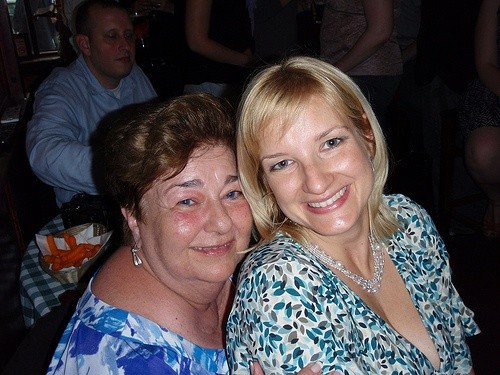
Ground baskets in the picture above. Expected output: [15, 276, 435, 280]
[39, 223, 108, 285]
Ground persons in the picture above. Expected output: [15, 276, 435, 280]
[320, 0, 406, 191]
[174, 0, 254, 100]
[45, 93, 341, 375]
[462, 0, 500, 240]
[225, 57, 481, 375]
[26, 0, 160, 210]
[13, 0, 59, 53]
[0, 0, 83, 180]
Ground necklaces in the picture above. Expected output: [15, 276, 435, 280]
[289, 206, 384, 294]
